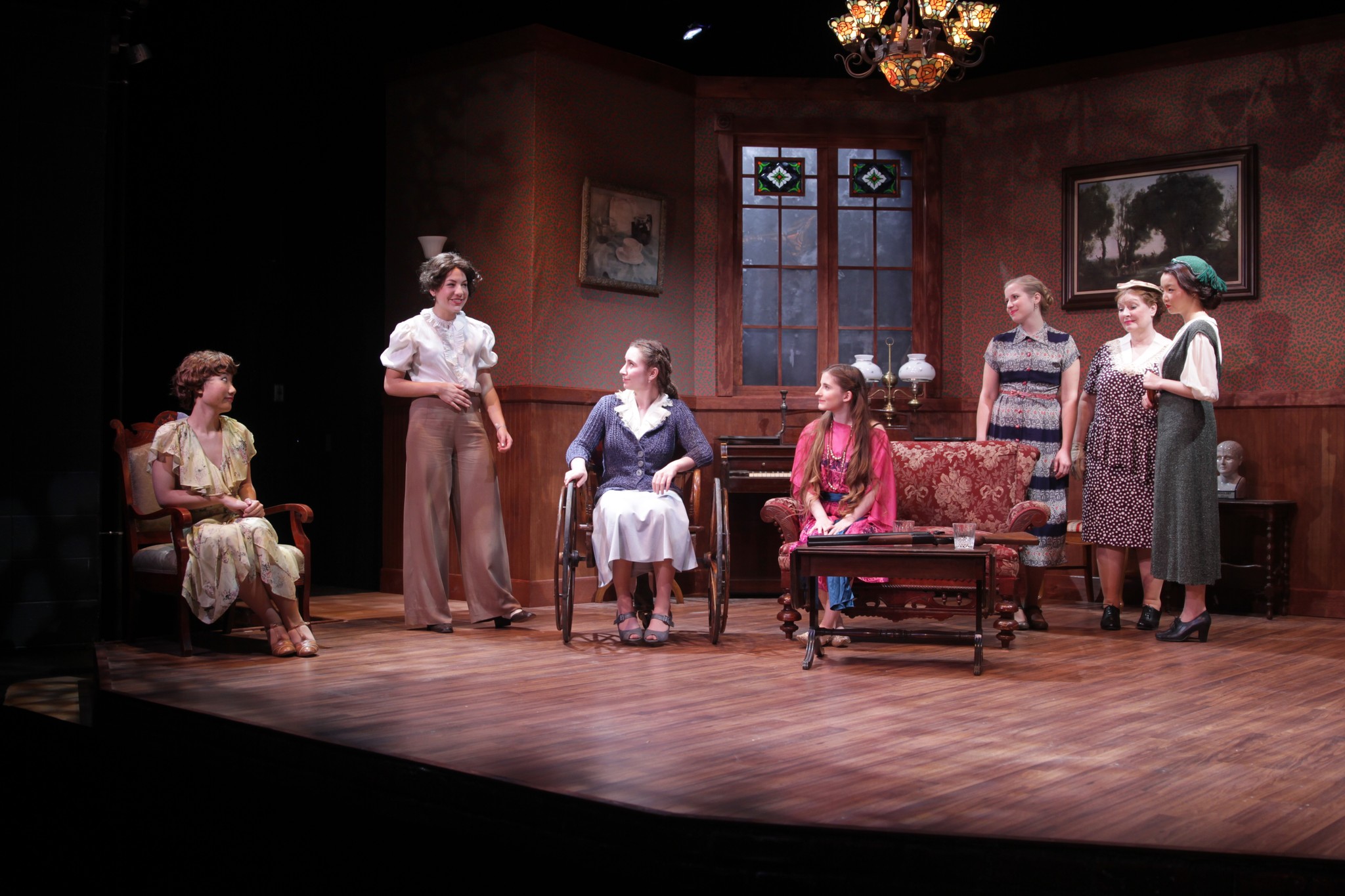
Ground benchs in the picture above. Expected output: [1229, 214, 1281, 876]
[760, 440, 1053, 651]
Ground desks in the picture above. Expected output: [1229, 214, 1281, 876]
[1046, 532, 1093, 602]
[788, 545, 995, 674]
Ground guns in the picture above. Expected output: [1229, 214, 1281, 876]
[807, 530, 1040, 547]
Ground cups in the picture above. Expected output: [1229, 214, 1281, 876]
[893, 521, 915, 533]
[952, 523, 977, 550]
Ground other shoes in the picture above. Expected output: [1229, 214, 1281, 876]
[831, 628, 851, 647]
[1025, 605, 1048, 630]
[796, 627, 830, 646]
[1013, 605, 1029, 629]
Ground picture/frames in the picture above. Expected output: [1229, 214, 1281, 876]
[1051, 141, 1261, 312]
[575, 171, 670, 299]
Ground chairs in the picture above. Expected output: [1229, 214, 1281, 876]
[107, 409, 316, 659]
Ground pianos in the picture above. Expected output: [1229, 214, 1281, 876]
[719, 441, 796, 594]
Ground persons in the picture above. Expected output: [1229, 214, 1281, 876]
[1070, 279, 1174, 631]
[564, 340, 713, 643]
[380, 252, 537, 634]
[783, 363, 897, 647]
[1216, 439, 1247, 499]
[977, 274, 1080, 631]
[1141, 255, 1227, 642]
[147, 350, 319, 657]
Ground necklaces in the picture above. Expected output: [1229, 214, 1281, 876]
[826, 419, 855, 490]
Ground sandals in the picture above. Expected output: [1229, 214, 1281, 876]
[286, 620, 318, 656]
[260, 623, 296, 656]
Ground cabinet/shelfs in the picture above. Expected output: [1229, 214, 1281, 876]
[1216, 499, 1300, 623]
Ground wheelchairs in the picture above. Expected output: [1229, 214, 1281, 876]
[552, 452, 730, 645]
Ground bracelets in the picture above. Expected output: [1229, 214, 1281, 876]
[496, 425, 507, 432]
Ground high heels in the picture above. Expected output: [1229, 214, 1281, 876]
[614, 610, 643, 641]
[427, 623, 454, 633]
[1136, 606, 1161, 629]
[1155, 611, 1212, 643]
[644, 610, 674, 643]
[495, 610, 536, 628]
[1101, 603, 1122, 629]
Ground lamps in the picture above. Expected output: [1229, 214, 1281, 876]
[824, 0, 1001, 96]
[846, 351, 937, 426]
